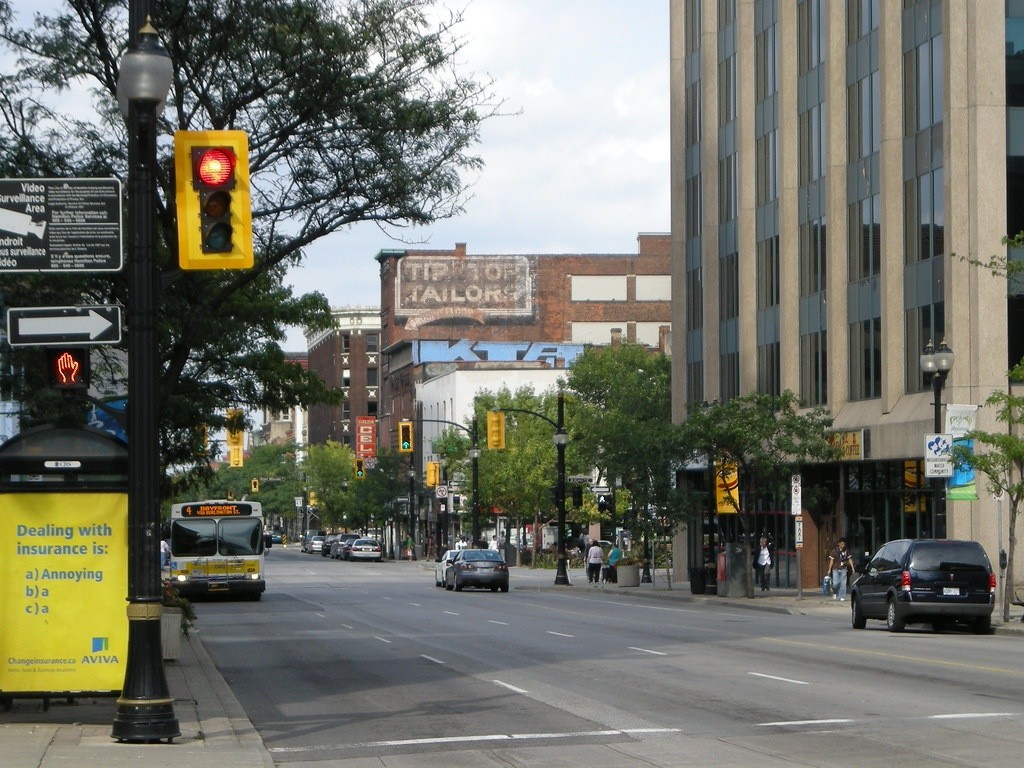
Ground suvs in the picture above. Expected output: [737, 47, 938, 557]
[322, 533, 360, 559]
[853, 538, 998, 632]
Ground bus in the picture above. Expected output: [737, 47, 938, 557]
[167, 499, 274, 600]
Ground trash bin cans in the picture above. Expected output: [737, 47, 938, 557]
[498, 543, 516, 564]
[400, 541, 408, 560]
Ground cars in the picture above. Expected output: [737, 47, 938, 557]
[342, 538, 381, 561]
[262, 524, 282, 542]
[434, 551, 460, 586]
[446, 550, 510, 593]
[308, 535, 325, 553]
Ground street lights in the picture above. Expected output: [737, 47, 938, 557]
[109, 22, 181, 744]
[921, 335, 954, 537]
[554, 427, 571, 585]
[407, 467, 417, 561]
[470, 443, 481, 549]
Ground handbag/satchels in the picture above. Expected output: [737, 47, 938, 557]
[164, 547, 169, 554]
[823, 575, 831, 595]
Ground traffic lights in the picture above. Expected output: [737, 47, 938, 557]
[173, 132, 255, 268]
[399, 421, 414, 453]
[252, 480, 258, 492]
[354, 459, 365, 478]
[50, 349, 89, 391]
[228, 489, 234, 500]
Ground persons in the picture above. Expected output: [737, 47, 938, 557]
[426, 531, 439, 561]
[406, 533, 414, 561]
[753, 537, 774, 591]
[161, 536, 169, 568]
[455, 535, 528, 556]
[827, 538, 855, 601]
[587, 540, 622, 584]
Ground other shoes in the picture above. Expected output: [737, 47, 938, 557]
[832, 594, 837, 601]
[761, 587, 769, 591]
[839, 597, 846, 602]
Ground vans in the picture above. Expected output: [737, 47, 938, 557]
[299, 529, 326, 552]
